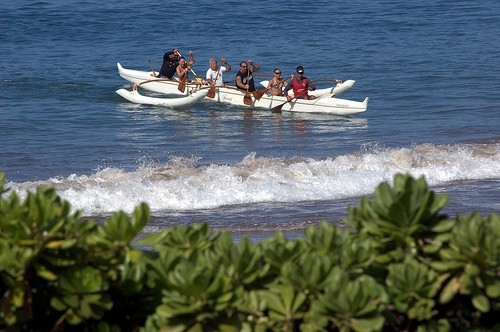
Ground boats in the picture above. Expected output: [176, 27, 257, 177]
[115, 62, 369, 117]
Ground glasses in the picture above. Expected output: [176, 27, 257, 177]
[241, 66, 247, 68]
[173, 54, 179, 56]
[275, 72, 281, 74]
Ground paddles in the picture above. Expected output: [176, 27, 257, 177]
[268, 87, 316, 114]
[308, 79, 343, 84]
[206, 57, 225, 98]
[177, 49, 192, 93]
[252, 73, 296, 99]
[173, 48, 204, 81]
[242, 57, 253, 106]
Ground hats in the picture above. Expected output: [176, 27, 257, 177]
[296, 66, 304, 73]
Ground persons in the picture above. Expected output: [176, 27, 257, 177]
[206, 57, 231, 87]
[156, 48, 180, 82]
[235, 59, 260, 92]
[267, 68, 294, 96]
[176, 50, 195, 83]
[284, 66, 316, 102]
